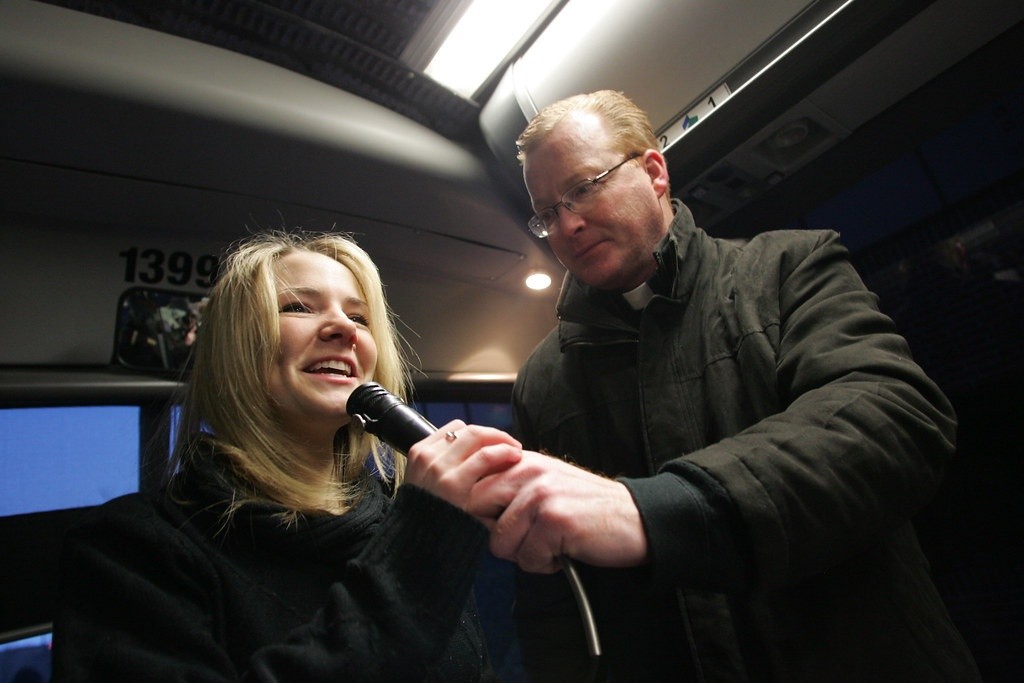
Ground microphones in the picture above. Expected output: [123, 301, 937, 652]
[345, 381, 439, 461]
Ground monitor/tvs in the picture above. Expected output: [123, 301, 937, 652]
[0, 378, 518, 683]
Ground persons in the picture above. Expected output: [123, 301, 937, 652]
[465, 89, 985, 683]
[51, 230, 523, 683]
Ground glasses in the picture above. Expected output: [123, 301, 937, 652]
[527, 154, 643, 239]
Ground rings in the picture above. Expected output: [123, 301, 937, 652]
[446, 431, 459, 439]
